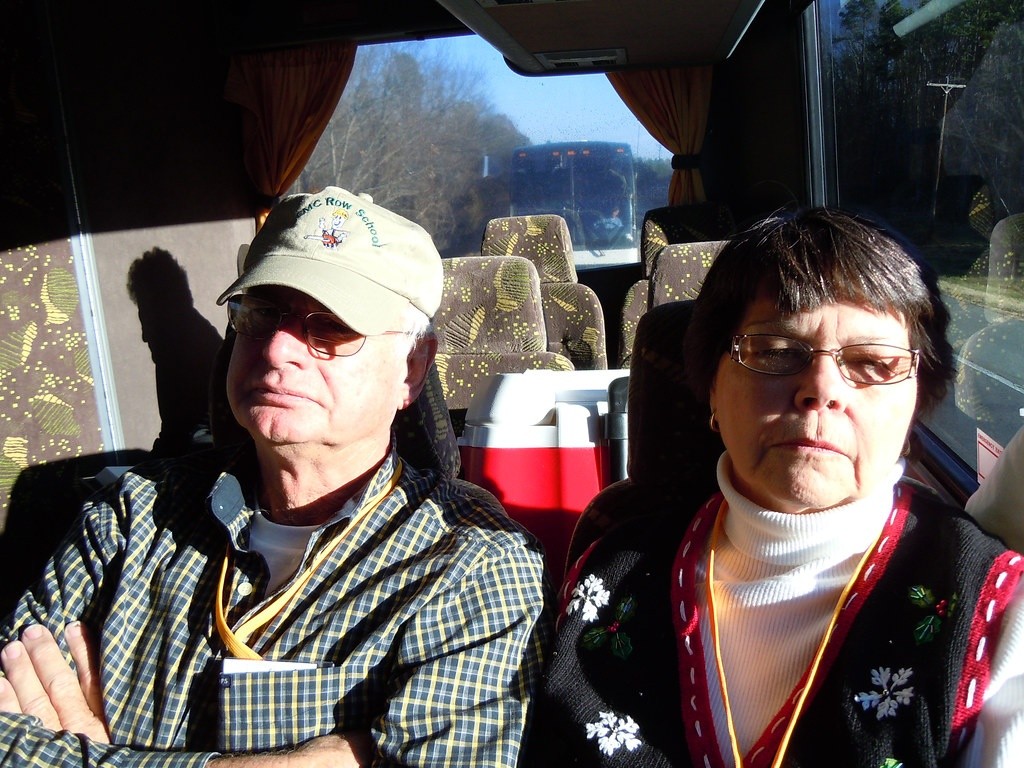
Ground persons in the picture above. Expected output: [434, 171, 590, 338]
[591, 206, 632, 241]
[525, 208, 1024, 768]
[0, 187, 556, 768]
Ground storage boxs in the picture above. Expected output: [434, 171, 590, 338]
[455, 368, 632, 595]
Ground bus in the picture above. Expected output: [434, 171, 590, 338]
[509, 142, 637, 252]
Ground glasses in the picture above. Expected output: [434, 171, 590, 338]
[725, 333, 920, 385]
[228, 295, 415, 357]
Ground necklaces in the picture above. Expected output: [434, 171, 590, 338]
[706, 497, 876, 768]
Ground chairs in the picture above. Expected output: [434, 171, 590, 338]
[389, 352, 506, 515]
[652, 240, 728, 311]
[481, 216, 608, 370]
[565, 300, 712, 577]
[621, 203, 732, 369]
[432, 256, 575, 435]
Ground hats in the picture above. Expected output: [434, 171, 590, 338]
[216, 185, 444, 337]
